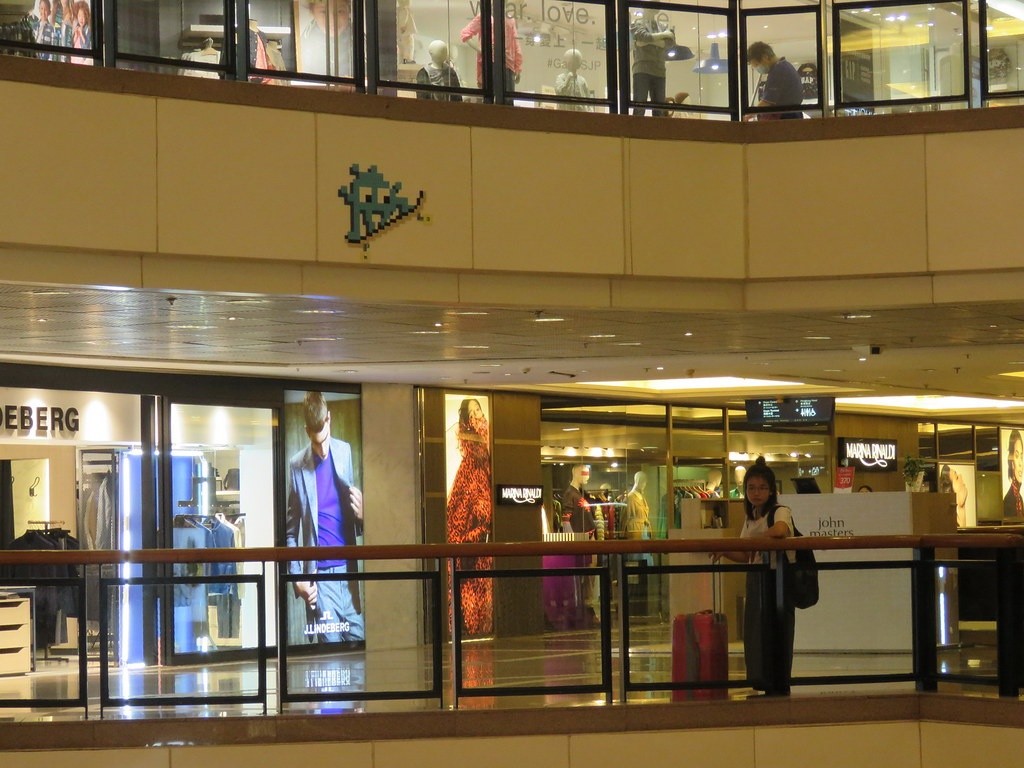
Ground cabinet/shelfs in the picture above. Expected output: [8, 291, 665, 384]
[0, 586, 37, 676]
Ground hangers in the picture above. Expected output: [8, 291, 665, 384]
[202, 37, 218, 55]
[174, 512, 240, 527]
[673, 478, 713, 493]
[554, 488, 614, 502]
[27, 521, 65, 537]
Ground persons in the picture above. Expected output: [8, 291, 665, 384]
[446, 396, 491, 630]
[555, 48, 593, 113]
[245, 19, 290, 86]
[284, 390, 366, 642]
[939, 465, 970, 530]
[705, 468, 723, 498]
[631, 0, 675, 117]
[299, 0, 351, 76]
[415, 40, 463, 103]
[620, 471, 652, 605]
[858, 483, 874, 494]
[561, 465, 597, 610]
[730, 465, 749, 500]
[459, 8, 523, 106]
[34, 0, 93, 67]
[744, 41, 804, 122]
[709, 457, 818, 701]
[1001, 428, 1024, 521]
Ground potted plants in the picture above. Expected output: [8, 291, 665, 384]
[900, 458, 927, 492]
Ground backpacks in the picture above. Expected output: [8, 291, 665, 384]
[767, 505, 819, 610]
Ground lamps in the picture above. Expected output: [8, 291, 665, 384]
[694, 43, 729, 74]
[665, 44, 694, 62]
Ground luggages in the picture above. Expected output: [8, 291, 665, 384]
[672, 554, 729, 701]
